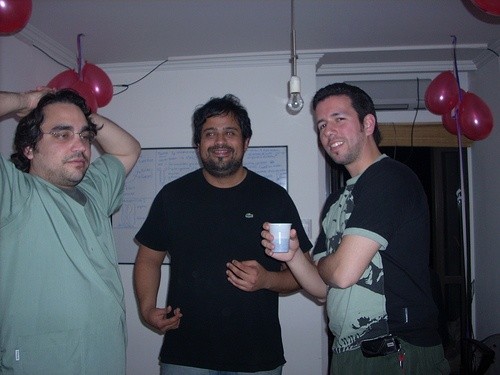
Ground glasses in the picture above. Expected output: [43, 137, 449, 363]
[43, 128, 96, 144]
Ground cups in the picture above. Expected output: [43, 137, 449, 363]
[268, 223, 292, 253]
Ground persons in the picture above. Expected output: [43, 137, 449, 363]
[133, 93, 313, 375]
[261, 82, 446, 375]
[0, 87, 141, 375]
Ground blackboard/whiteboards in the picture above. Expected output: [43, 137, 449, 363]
[111, 144, 289, 265]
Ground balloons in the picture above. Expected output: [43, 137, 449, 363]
[442, 89, 465, 136]
[458, 91, 494, 141]
[45, 62, 112, 113]
[471, 0, 500, 16]
[425, 70, 458, 116]
[0, 0, 32, 36]
